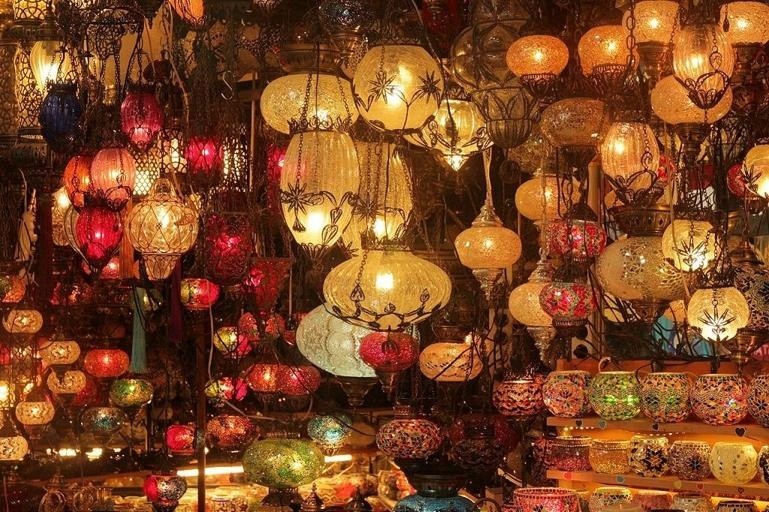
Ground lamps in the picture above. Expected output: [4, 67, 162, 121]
[0, 0, 769, 512]
